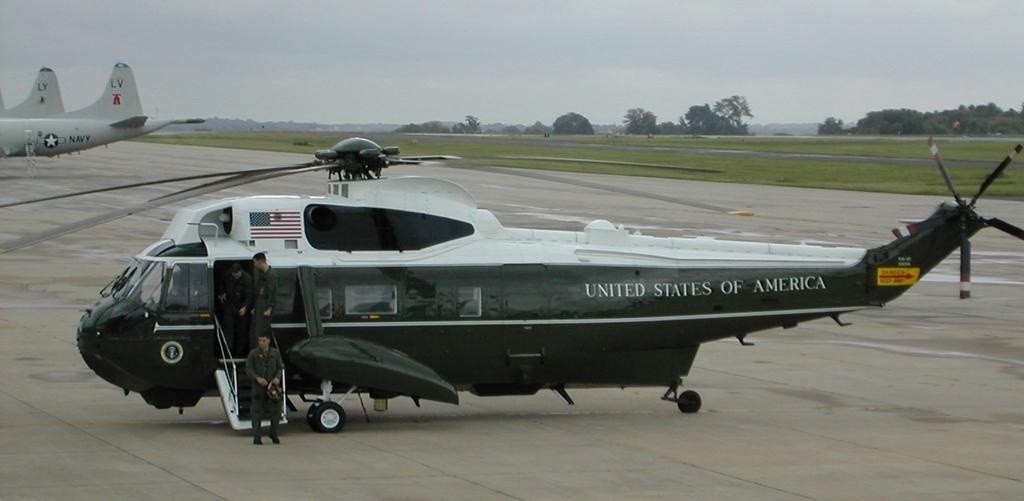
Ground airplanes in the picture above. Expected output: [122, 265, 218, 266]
[0, 63, 205, 158]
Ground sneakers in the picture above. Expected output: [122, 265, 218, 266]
[268, 432, 282, 444]
[252, 434, 264, 445]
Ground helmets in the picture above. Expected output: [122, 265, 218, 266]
[264, 380, 283, 403]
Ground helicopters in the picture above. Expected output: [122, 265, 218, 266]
[0, 135, 1024, 434]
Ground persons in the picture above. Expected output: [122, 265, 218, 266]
[220, 253, 284, 445]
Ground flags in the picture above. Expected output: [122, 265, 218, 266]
[249, 212, 303, 240]
